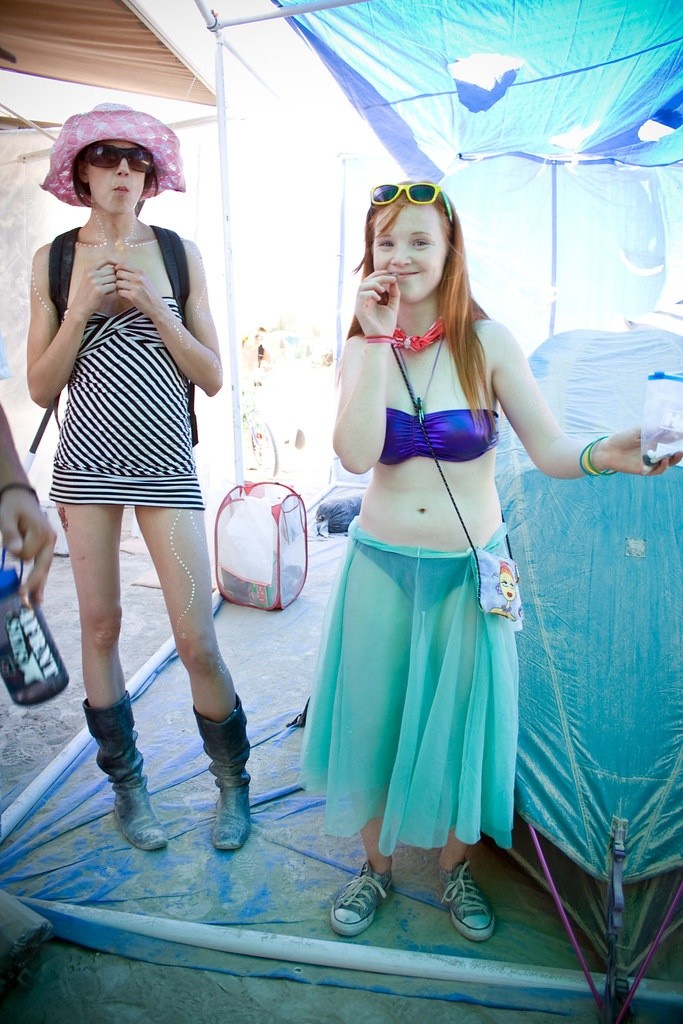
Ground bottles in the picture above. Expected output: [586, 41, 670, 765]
[0, 547, 70, 706]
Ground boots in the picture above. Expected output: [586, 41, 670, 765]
[82, 690, 168, 850]
[194, 691, 251, 850]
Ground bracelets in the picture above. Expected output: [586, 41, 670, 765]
[364, 334, 394, 345]
[0, 483, 40, 504]
[578, 436, 619, 478]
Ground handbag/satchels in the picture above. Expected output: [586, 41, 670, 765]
[467, 547, 524, 623]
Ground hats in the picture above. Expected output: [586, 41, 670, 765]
[39, 103, 186, 207]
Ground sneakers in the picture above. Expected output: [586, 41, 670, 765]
[331, 858, 393, 936]
[439, 856, 495, 941]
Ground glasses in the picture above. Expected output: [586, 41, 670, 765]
[89, 144, 154, 173]
[370, 183, 453, 224]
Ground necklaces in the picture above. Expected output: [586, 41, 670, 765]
[395, 315, 446, 428]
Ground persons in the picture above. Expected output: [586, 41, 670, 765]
[302, 182, 683, 944]
[0, 408, 57, 601]
[26, 105, 252, 853]
